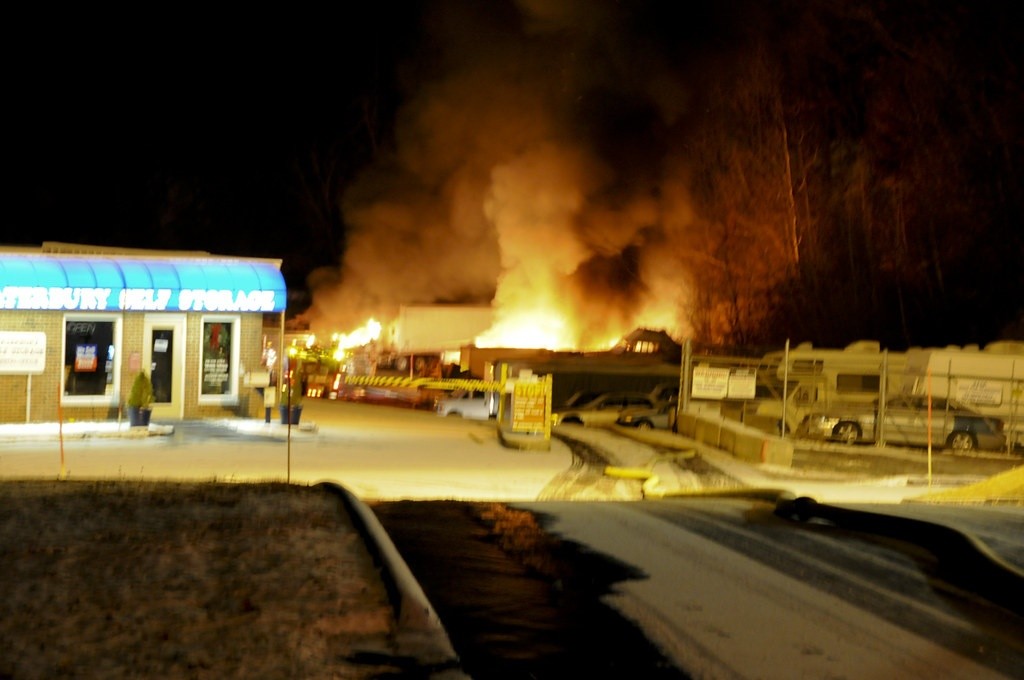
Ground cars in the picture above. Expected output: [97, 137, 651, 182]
[551, 395, 655, 428]
[618, 400, 679, 429]
[811, 395, 1007, 451]
[437, 389, 501, 420]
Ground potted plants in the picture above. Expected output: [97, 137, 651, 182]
[279, 372, 303, 425]
[127, 369, 154, 426]
